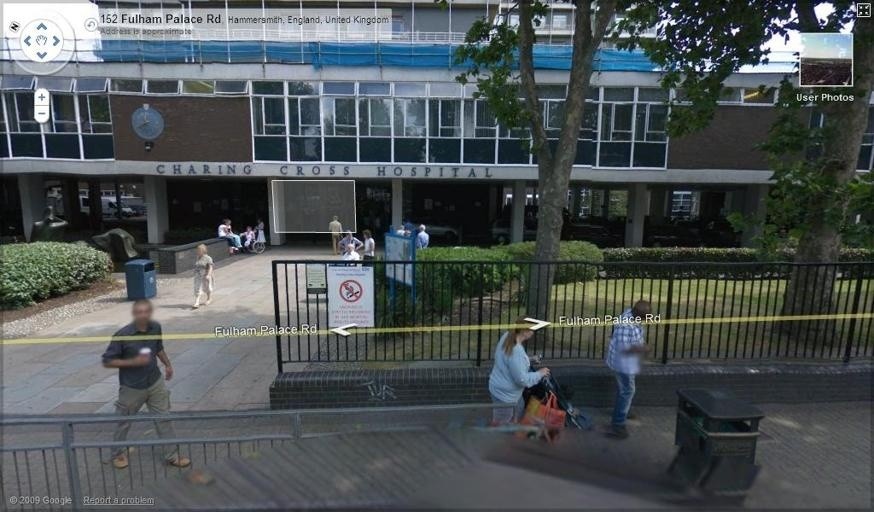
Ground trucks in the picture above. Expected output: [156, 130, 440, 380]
[47, 193, 147, 221]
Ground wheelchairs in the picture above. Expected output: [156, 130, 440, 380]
[241, 228, 265, 255]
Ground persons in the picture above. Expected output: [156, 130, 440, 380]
[217, 219, 239, 251]
[603, 299, 654, 438]
[100, 296, 191, 469]
[190, 243, 213, 308]
[323, 214, 430, 270]
[239, 225, 255, 252]
[485, 317, 552, 427]
[181, 469, 216, 487]
[226, 218, 242, 248]
[253, 218, 266, 249]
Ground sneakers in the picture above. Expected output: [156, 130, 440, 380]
[113, 452, 128, 469]
[171, 455, 190, 467]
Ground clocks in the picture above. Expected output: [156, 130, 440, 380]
[128, 103, 165, 143]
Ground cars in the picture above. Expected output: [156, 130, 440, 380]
[485, 206, 738, 249]
[404, 216, 464, 242]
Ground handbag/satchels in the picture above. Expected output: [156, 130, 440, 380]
[514, 391, 566, 454]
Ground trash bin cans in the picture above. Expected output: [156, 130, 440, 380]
[665, 387, 765, 504]
[123, 259, 156, 300]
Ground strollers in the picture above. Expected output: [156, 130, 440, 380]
[522, 358, 593, 444]
